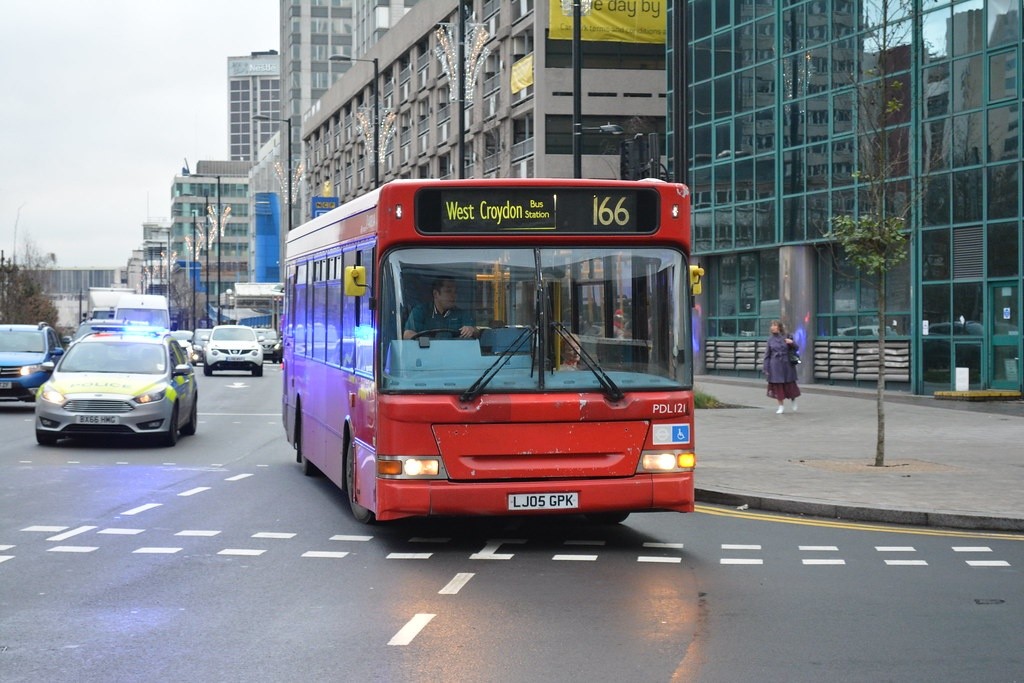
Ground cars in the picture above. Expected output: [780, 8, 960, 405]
[839, 321, 1018, 378]
[35, 319, 197, 445]
[170, 325, 284, 376]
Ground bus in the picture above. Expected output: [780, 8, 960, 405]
[283, 178, 704, 525]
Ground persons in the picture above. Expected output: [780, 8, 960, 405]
[553, 333, 582, 371]
[763, 319, 799, 414]
[577, 309, 632, 340]
[401, 275, 480, 340]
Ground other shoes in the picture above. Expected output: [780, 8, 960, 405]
[776, 406, 784, 414]
[792, 399, 797, 410]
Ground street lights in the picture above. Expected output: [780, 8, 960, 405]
[151, 230, 170, 317]
[328, 54, 379, 192]
[572, 0, 625, 179]
[190, 174, 220, 326]
[252, 115, 292, 231]
[145, 241, 162, 295]
[183, 193, 209, 329]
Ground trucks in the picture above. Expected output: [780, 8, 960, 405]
[82, 288, 170, 328]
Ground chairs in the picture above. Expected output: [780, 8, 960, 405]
[480, 328, 534, 356]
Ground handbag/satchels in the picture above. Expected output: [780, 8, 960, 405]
[787, 334, 800, 365]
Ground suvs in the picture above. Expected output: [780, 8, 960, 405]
[0, 321, 64, 403]
